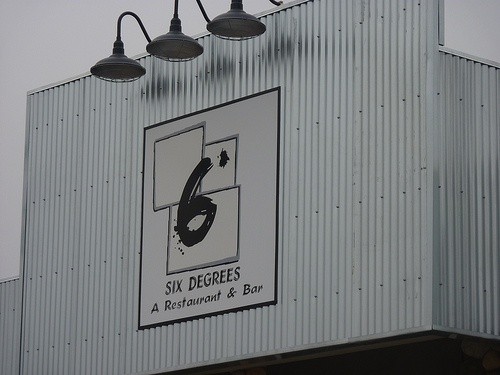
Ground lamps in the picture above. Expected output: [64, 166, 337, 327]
[146, 0, 211, 62]
[90, 11, 152, 83]
[206, 0, 283, 41]
